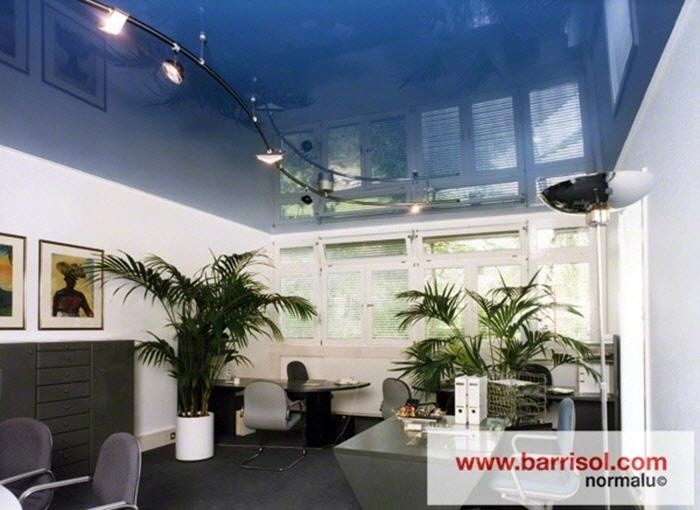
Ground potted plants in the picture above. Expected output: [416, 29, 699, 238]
[80, 246, 318, 462]
[397, 265, 603, 415]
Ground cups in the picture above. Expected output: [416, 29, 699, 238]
[234, 378, 239, 385]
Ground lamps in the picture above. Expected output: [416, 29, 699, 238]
[256, 153, 284, 165]
[95, 8, 131, 35]
[162, 43, 185, 86]
[587, 208, 610, 228]
[410, 205, 421, 213]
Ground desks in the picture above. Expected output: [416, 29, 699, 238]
[213, 376, 371, 435]
[547, 393, 619, 430]
[412, 383, 454, 413]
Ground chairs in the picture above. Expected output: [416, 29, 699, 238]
[239, 383, 308, 473]
[19, 434, 141, 510]
[0, 418, 54, 509]
[379, 378, 410, 420]
[517, 365, 552, 425]
[494, 399, 582, 509]
[287, 361, 309, 400]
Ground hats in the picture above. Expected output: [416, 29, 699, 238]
[57, 262, 86, 278]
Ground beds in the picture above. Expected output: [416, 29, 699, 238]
[0, 484, 23, 510]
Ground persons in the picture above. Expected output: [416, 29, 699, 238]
[52, 261, 94, 318]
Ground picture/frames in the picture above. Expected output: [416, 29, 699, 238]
[37, 238, 106, 331]
[0, 232, 26, 331]
